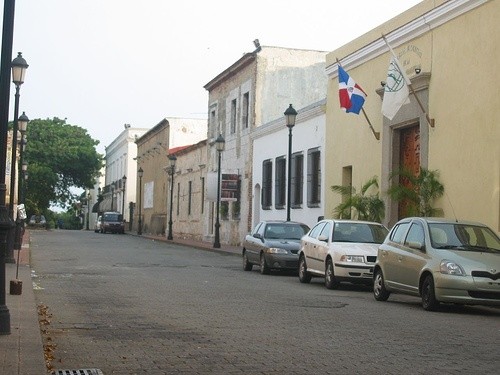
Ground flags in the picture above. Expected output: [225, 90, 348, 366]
[338, 65, 367, 114]
[381, 40, 412, 120]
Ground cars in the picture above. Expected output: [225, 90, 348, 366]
[99, 211, 125, 234]
[94, 216, 103, 233]
[370, 216, 500, 310]
[296, 219, 393, 290]
[242, 219, 310, 275]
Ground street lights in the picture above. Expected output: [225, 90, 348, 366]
[97, 185, 100, 213]
[282, 103, 298, 221]
[5, 50, 29, 265]
[121, 174, 127, 216]
[111, 182, 117, 212]
[15, 111, 29, 248]
[212, 134, 226, 248]
[166, 154, 177, 240]
[136, 167, 146, 234]
